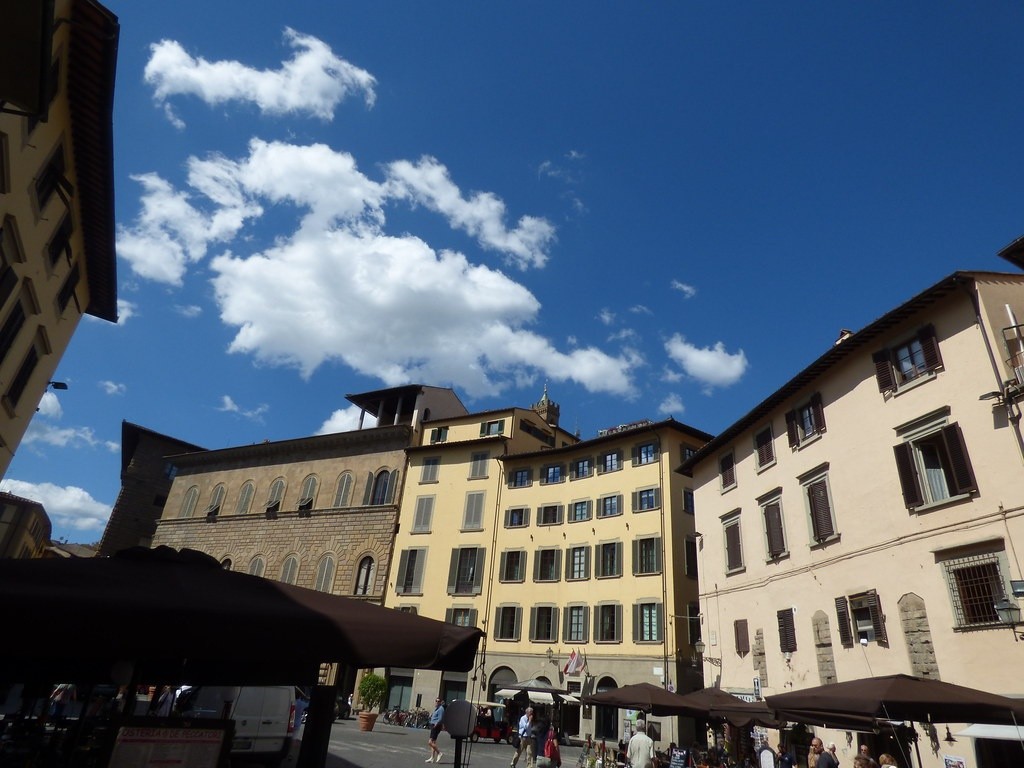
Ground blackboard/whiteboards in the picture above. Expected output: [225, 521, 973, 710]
[669, 748, 689, 768]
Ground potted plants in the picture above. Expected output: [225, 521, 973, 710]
[357, 673, 387, 731]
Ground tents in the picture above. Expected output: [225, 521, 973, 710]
[0, 544, 479, 768]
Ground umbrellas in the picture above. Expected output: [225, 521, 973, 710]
[495, 679, 580, 707]
[585, 674, 1024, 750]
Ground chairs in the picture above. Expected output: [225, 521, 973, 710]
[0, 712, 137, 768]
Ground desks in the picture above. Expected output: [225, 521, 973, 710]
[587, 757, 614, 768]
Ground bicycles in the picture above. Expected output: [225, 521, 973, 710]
[383, 705, 430, 729]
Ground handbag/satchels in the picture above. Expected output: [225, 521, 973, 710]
[544, 729, 560, 762]
[50, 700, 57, 714]
[512, 735, 521, 749]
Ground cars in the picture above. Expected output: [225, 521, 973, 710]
[295, 686, 351, 723]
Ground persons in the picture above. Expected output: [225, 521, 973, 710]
[510, 707, 534, 768]
[425, 698, 444, 763]
[750, 737, 797, 768]
[627, 719, 656, 768]
[289, 693, 309, 760]
[854, 745, 897, 768]
[347, 694, 353, 716]
[669, 742, 719, 768]
[545, 730, 562, 768]
[808, 737, 839, 768]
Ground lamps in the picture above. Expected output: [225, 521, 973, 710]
[943, 724, 957, 746]
[995, 580, 1024, 642]
[691, 531, 703, 538]
[979, 391, 1006, 408]
[48, 381, 68, 390]
[695, 636, 722, 667]
[546, 647, 559, 664]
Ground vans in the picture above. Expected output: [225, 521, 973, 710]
[172, 685, 296, 762]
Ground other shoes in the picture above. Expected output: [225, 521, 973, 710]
[435, 752, 445, 762]
[425, 758, 435, 763]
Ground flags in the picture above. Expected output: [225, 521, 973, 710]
[563, 650, 589, 676]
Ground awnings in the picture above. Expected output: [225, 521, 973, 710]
[951, 723, 1024, 742]
